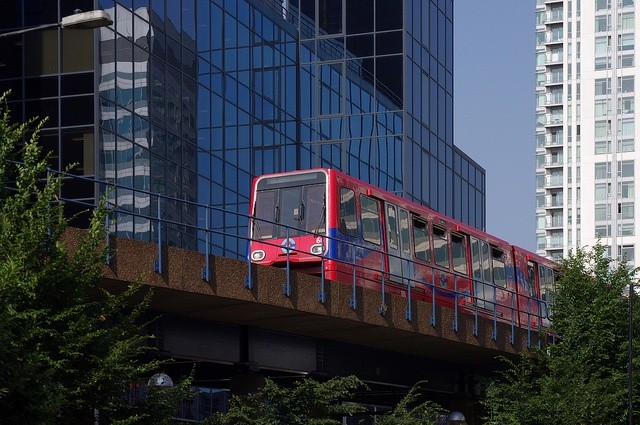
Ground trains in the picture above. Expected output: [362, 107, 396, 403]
[246, 167, 565, 335]
